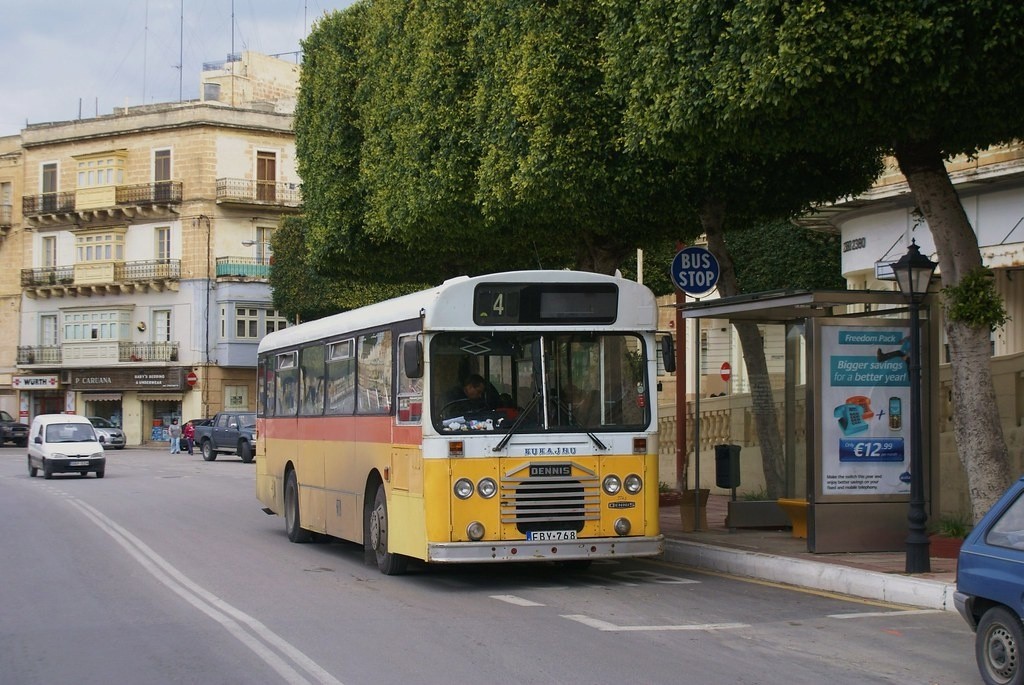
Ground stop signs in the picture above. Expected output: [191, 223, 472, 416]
[719, 362, 732, 381]
[187, 373, 197, 386]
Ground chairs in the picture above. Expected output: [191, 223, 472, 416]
[72, 430, 80, 440]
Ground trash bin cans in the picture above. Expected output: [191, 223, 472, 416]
[714, 444, 741, 488]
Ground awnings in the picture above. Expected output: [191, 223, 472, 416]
[136, 392, 182, 401]
[80, 393, 122, 401]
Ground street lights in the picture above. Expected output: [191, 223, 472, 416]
[890, 237, 939, 574]
[192, 214, 210, 419]
[241, 240, 300, 325]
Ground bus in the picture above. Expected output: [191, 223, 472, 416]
[255, 270, 677, 576]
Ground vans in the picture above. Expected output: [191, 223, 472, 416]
[27, 414, 106, 479]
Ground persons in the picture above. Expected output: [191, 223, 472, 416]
[185, 422, 194, 455]
[436, 374, 485, 420]
[169, 418, 182, 454]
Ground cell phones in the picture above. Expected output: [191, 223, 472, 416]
[888, 397, 901, 431]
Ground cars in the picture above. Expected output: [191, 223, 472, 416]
[170, 419, 209, 451]
[64, 417, 126, 450]
[0, 411, 30, 447]
[953, 476, 1024, 685]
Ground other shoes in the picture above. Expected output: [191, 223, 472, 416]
[188, 452, 193, 455]
[171, 451, 180, 454]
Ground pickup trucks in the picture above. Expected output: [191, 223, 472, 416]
[194, 411, 263, 463]
